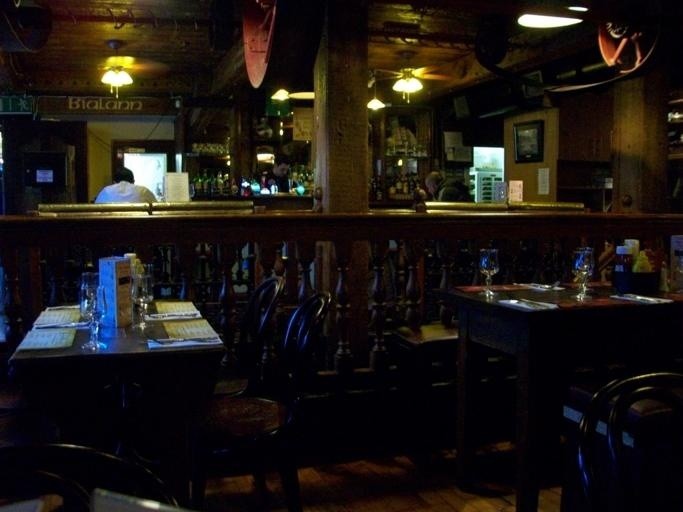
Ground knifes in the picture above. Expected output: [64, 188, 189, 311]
[516, 298, 547, 309]
[47, 307, 80, 311]
[35, 322, 89, 330]
[148, 313, 196, 319]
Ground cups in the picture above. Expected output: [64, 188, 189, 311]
[241, 182, 250, 197]
[270, 184, 278, 195]
[494, 181, 509, 204]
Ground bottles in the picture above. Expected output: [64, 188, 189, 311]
[375, 187, 384, 201]
[192, 169, 230, 196]
[123, 252, 145, 276]
[614, 239, 662, 272]
[260, 171, 268, 188]
[387, 172, 414, 195]
[411, 143, 427, 157]
[287, 166, 313, 190]
[658, 261, 670, 292]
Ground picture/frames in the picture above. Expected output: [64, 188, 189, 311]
[513, 120, 544, 163]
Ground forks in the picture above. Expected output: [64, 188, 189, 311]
[532, 280, 560, 290]
[145, 334, 219, 345]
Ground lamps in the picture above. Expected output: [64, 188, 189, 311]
[515, 0, 583, 30]
[522, 70, 544, 99]
[97, 49, 136, 100]
[392, 53, 424, 104]
[367, 83, 385, 112]
[271, 88, 315, 102]
[194, 16, 215, 32]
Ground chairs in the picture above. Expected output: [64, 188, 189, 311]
[0, 441, 192, 512]
[205, 275, 287, 402]
[560, 370, 682, 512]
[214, 290, 332, 444]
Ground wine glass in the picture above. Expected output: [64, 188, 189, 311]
[568, 246, 595, 300]
[478, 247, 498, 296]
[129, 272, 154, 330]
[190, 142, 226, 156]
[78, 271, 110, 351]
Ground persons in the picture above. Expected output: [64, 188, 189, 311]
[94, 166, 157, 203]
[261, 154, 295, 189]
[421, 170, 473, 202]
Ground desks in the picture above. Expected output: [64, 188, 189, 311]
[433, 282, 674, 453]
[8, 295, 226, 467]
